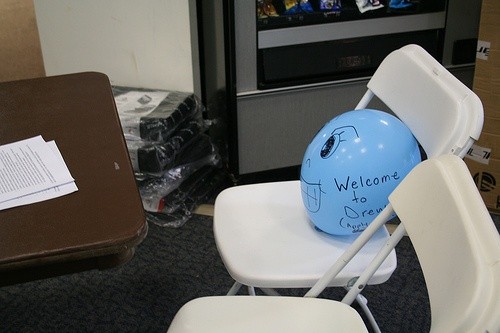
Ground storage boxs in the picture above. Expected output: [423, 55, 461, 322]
[111, 85, 214, 227]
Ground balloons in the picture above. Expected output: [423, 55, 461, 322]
[300, 109, 422, 235]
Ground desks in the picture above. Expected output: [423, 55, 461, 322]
[0, 71, 149, 288]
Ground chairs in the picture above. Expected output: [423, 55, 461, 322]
[166, 44, 500, 333]
